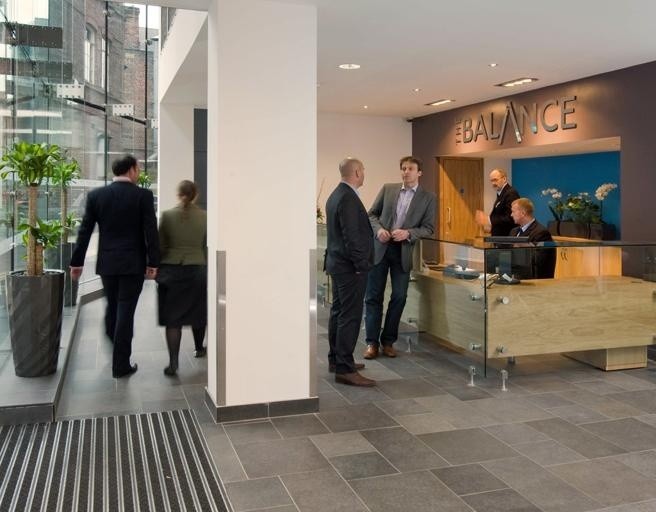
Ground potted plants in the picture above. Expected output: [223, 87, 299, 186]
[0, 136, 84, 378]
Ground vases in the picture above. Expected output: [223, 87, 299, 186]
[547, 219, 617, 240]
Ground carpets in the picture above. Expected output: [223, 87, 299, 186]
[0, 409, 236, 511]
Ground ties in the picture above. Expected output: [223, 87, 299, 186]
[516, 229, 523, 234]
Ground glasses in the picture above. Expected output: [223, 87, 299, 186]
[491, 176, 503, 182]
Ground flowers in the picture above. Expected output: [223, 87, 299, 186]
[541, 181, 618, 221]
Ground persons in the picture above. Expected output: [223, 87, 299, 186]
[154, 180, 206, 376]
[475, 167, 521, 241]
[507, 195, 553, 281]
[363, 156, 438, 359]
[322, 155, 378, 387]
[68, 154, 157, 381]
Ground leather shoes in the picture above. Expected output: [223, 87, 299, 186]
[330, 362, 364, 372]
[165, 361, 178, 375]
[381, 344, 396, 357]
[113, 363, 137, 377]
[335, 372, 375, 387]
[194, 346, 207, 357]
[363, 343, 378, 358]
